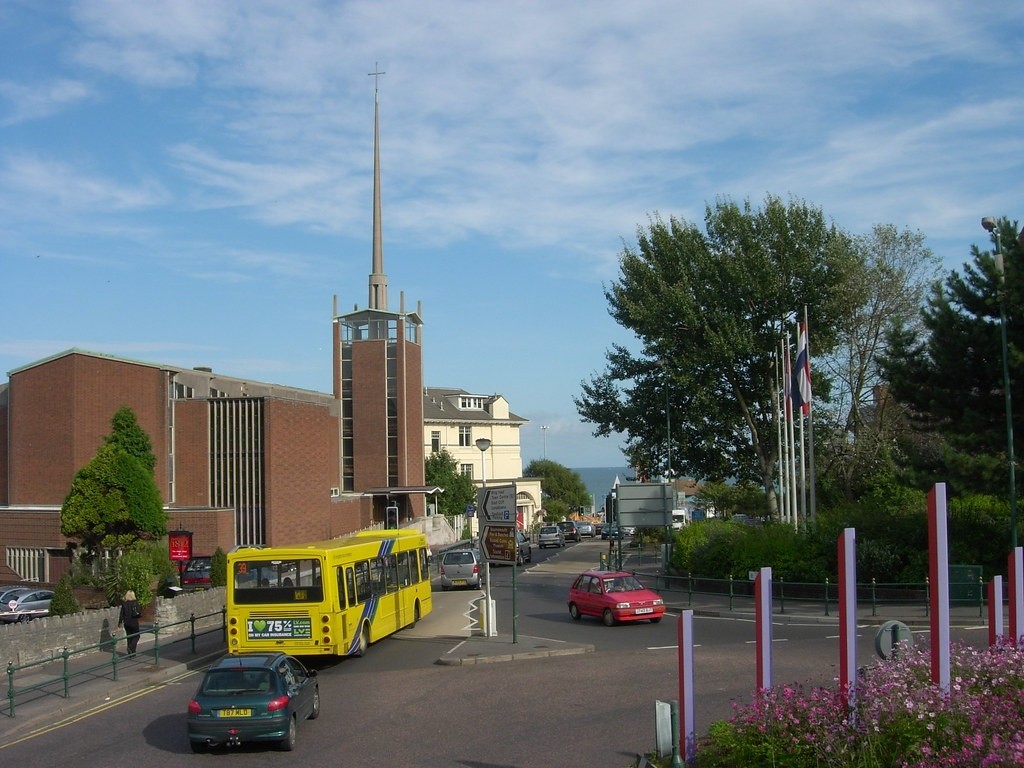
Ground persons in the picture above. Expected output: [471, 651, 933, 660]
[118, 590, 141, 659]
[284, 577, 293, 586]
[262, 578, 269, 587]
[316, 576, 321, 585]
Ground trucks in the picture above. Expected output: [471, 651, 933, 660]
[182, 555, 213, 590]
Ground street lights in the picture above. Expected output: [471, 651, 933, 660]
[475, 438, 492, 487]
[541, 425, 549, 460]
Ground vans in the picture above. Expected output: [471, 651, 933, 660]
[440, 550, 490, 590]
[671, 509, 692, 530]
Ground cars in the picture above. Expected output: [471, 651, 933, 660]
[0, 589, 55, 623]
[187, 651, 321, 753]
[539, 525, 565, 549]
[578, 522, 596, 538]
[600, 523, 622, 540]
[622, 526, 638, 537]
[566, 569, 666, 626]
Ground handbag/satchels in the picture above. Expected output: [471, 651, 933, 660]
[131, 600, 142, 618]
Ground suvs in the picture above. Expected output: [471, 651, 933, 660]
[558, 521, 581, 542]
[489, 532, 532, 567]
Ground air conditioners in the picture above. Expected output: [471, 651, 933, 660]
[331, 488, 339, 497]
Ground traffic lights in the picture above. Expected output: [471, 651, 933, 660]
[385, 507, 398, 530]
[580, 506, 585, 516]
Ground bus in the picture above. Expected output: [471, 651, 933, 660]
[226, 528, 433, 657]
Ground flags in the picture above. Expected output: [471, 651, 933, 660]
[784, 347, 792, 418]
[792, 322, 811, 415]
